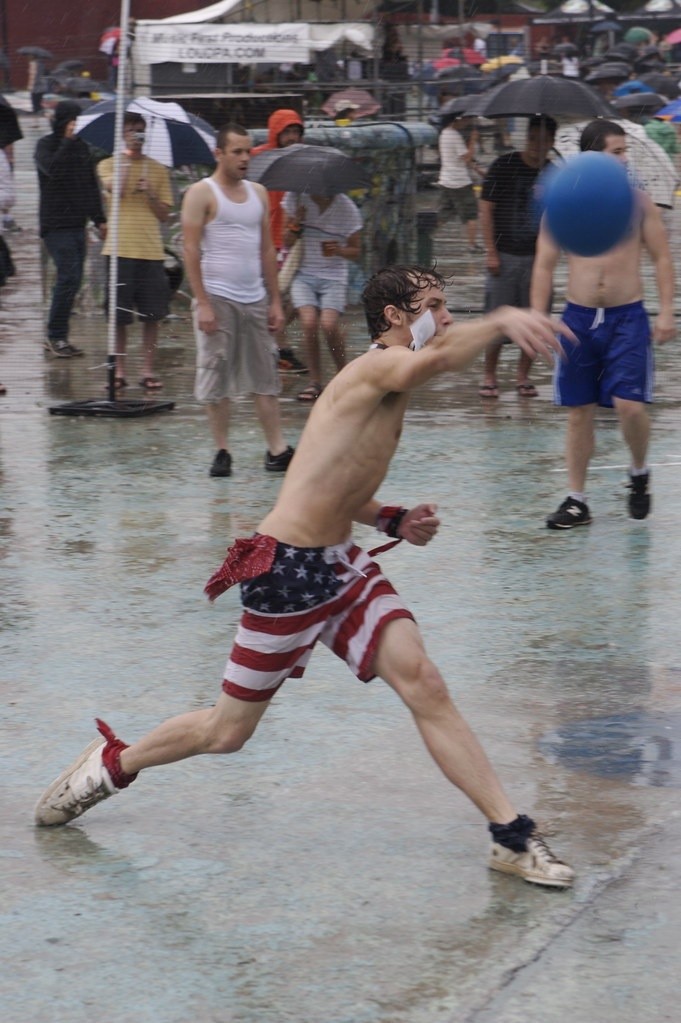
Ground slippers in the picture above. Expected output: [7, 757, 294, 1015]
[516, 381, 539, 397]
[480, 377, 497, 398]
[114, 375, 126, 389]
[140, 374, 165, 389]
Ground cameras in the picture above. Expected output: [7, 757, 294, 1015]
[133, 132, 144, 143]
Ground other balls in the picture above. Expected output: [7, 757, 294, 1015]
[545, 150, 633, 257]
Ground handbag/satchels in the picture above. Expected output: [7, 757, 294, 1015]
[276, 235, 305, 291]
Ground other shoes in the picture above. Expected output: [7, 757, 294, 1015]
[42, 335, 84, 358]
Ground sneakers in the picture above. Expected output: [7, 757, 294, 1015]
[627, 466, 650, 521]
[486, 824, 572, 888]
[209, 448, 230, 479]
[265, 445, 295, 471]
[277, 353, 311, 373]
[545, 497, 593, 527]
[34, 737, 123, 826]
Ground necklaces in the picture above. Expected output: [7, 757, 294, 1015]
[369, 343, 389, 350]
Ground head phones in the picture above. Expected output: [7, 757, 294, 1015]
[368, 339, 425, 352]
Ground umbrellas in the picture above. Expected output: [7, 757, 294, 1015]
[244, 143, 374, 206]
[317, 86, 382, 117]
[40, 60, 101, 92]
[71, 93, 221, 168]
[530, 0, 681, 24]
[0, 94, 23, 148]
[413, 20, 681, 116]
[15, 45, 54, 62]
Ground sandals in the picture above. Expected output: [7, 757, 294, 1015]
[298, 383, 320, 402]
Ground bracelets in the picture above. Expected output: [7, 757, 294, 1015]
[288, 223, 305, 236]
[376, 505, 402, 532]
[387, 507, 407, 537]
[149, 193, 158, 201]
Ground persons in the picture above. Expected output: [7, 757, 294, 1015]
[535, 37, 551, 60]
[250, 109, 304, 324]
[282, 184, 364, 402]
[0, 146, 16, 395]
[560, 54, 581, 80]
[34, 101, 175, 390]
[180, 123, 297, 478]
[332, 99, 361, 121]
[24, 52, 43, 112]
[551, 36, 579, 61]
[421, 114, 676, 529]
[38, 265, 579, 888]
